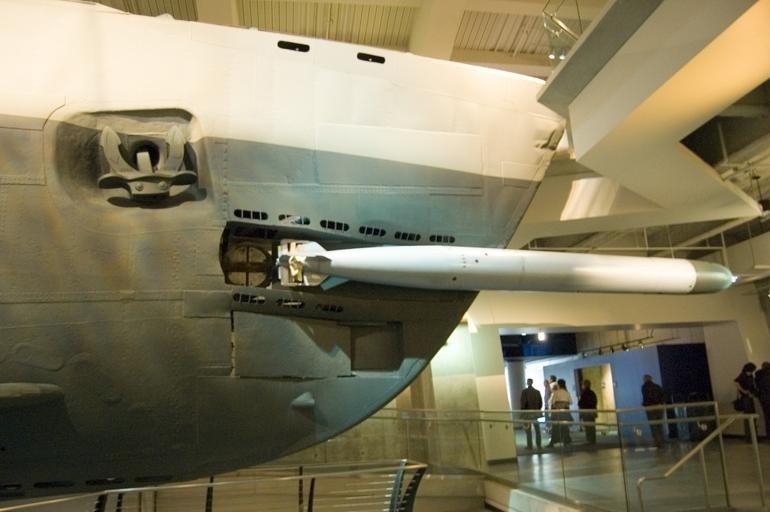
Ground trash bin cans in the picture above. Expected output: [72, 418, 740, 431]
[672, 407, 690, 441]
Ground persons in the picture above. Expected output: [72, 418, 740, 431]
[519, 378, 542, 450]
[543, 375, 574, 448]
[641, 374, 668, 449]
[734, 363, 760, 445]
[578, 379, 597, 445]
[754, 361, 770, 439]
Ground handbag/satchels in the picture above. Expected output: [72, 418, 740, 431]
[732, 399, 746, 411]
